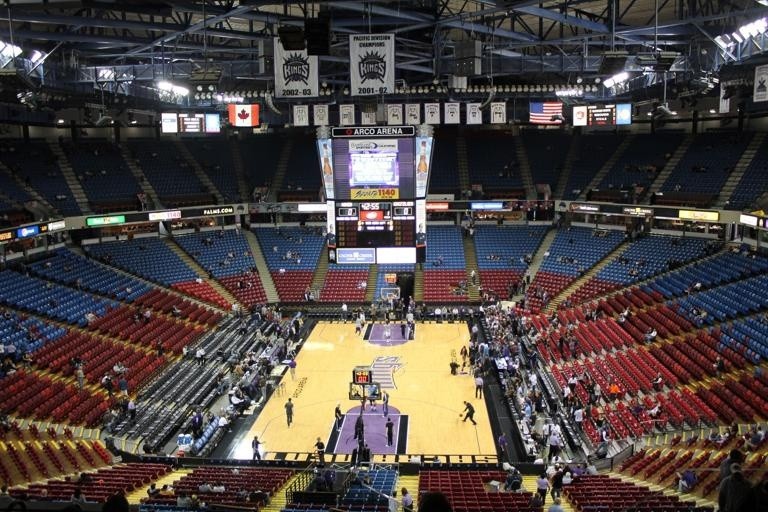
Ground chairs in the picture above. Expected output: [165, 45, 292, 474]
[0, 223, 768, 512]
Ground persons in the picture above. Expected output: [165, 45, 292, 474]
[0, 141, 768, 511]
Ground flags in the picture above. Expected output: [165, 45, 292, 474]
[227, 104, 259, 128]
[530, 101, 563, 125]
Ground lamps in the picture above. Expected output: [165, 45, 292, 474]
[0, 40, 245, 103]
[555, 0, 768, 98]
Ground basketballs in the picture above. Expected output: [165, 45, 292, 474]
[459, 413, 463, 417]
